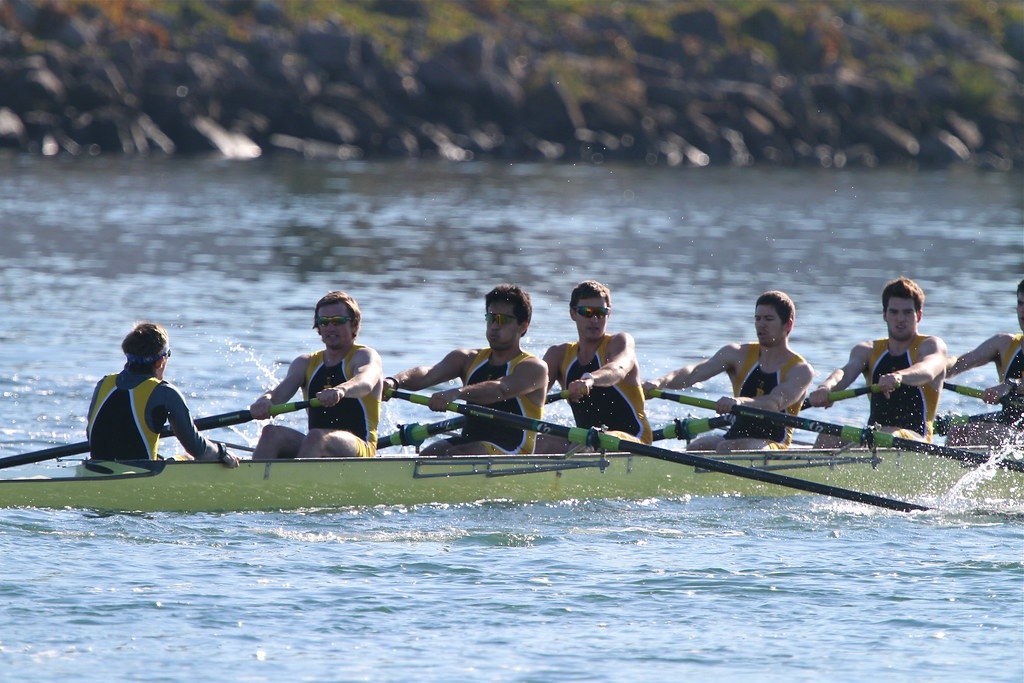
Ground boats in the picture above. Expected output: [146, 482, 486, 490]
[0, 424, 1024, 512]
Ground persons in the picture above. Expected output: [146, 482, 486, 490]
[640, 291, 814, 454]
[249, 290, 383, 459]
[945, 280, 1024, 446]
[85, 323, 241, 469]
[809, 276, 947, 450]
[383, 286, 548, 455]
[534, 281, 652, 455]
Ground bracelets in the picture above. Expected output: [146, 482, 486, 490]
[386, 377, 400, 390]
[580, 372, 595, 382]
[1005, 377, 1018, 396]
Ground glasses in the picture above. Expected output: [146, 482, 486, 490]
[485, 313, 517, 325]
[161, 350, 171, 356]
[572, 306, 612, 318]
[316, 315, 353, 326]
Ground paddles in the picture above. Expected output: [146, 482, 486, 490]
[0, 393, 342, 470]
[643, 388, 1024, 473]
[652, 384, 876, 441]
[933, 410, 1020, 437]
[383, 387, 928, 513]
[377, 384, 595, 450]
[943, 382, 1024, 409]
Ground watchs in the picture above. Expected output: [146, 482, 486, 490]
[215, 441, 226, 460]
